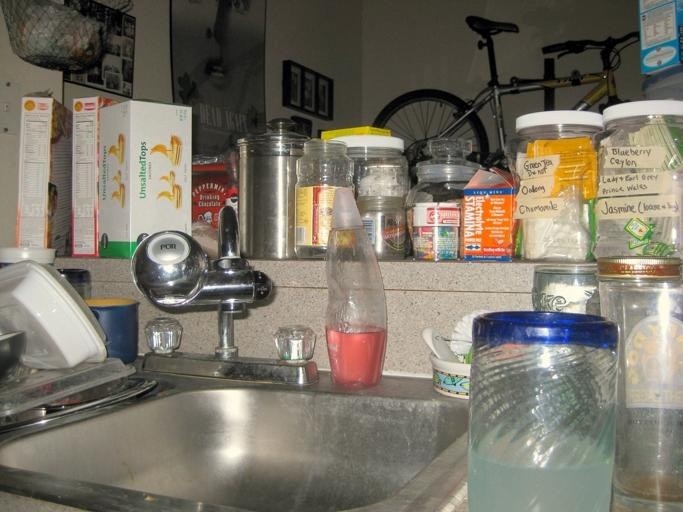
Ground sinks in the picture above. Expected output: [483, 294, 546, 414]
[0, 380, 468, 508]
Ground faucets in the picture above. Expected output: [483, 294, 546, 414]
[133, 206, 271, 358]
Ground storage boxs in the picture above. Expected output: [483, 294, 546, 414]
[16, 88, 194, 262]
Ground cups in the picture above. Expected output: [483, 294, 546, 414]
[84, 298, 139, 363]
[597, 280, 681, 507]
[0, 248, 57, 270]
[56, 268, 91, 300]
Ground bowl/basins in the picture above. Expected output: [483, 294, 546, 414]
[429, 353, 471, 399]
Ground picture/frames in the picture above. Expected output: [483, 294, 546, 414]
[282, 58, 335, 138]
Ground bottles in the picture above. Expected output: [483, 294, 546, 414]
[324, 188, 388, 386]
[514, 99, 682, 314]
[466, 310, 616, 511]
[235, 117, 309, 260]
[294, 135, 483, 260]
